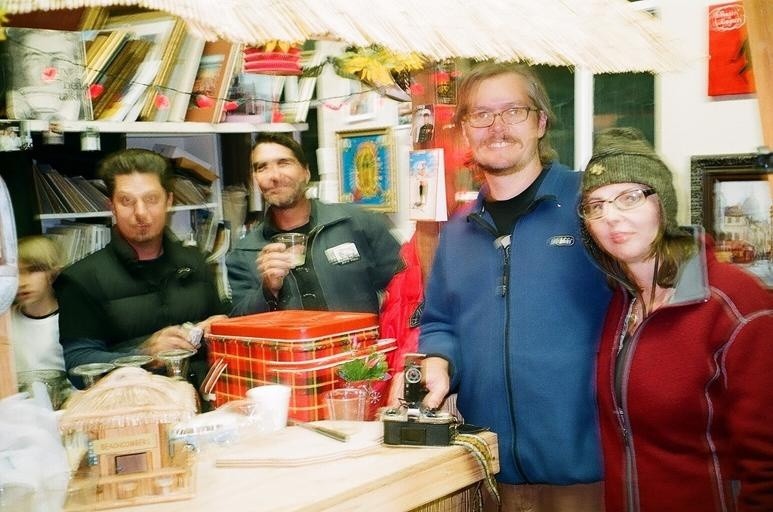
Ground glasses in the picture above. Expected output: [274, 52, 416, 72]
[460, 104, 541, 129]
[576, 188, 658, 219]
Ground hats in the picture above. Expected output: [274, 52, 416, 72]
[575, 126, 691, 240]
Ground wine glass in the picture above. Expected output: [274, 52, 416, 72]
[18, 348, 198, 410]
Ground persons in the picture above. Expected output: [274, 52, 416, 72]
[418, 113, 436, 144]
[51, 147, 234, 415]
[387, 64, 612, 512]
[8, 235, 77, 409]
[579, 128, 772, 512]
[224, 133, 405, 313]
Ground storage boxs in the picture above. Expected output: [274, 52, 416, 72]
[207, 307, 396, 425]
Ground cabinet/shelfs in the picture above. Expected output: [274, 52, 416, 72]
[0, 115, 309, 287]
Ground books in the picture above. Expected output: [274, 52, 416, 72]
[33, 146, 232, 272]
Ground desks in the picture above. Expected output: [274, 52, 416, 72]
[0, 411, 500, 512]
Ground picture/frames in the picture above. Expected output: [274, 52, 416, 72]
[104, 11, 188, 120]
[337, 125, 399, 215]
[690, 152, 773, 290]
[185, 39, 240, 120]
[81, 27, 128, 81]
[0, 4, 109, 27]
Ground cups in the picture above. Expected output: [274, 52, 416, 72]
[273, 232, 308, 266]
[326, 387, 367, 427]
[247, 383, 291, 427]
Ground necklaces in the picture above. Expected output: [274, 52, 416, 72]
[626, 305, 651, 336]
